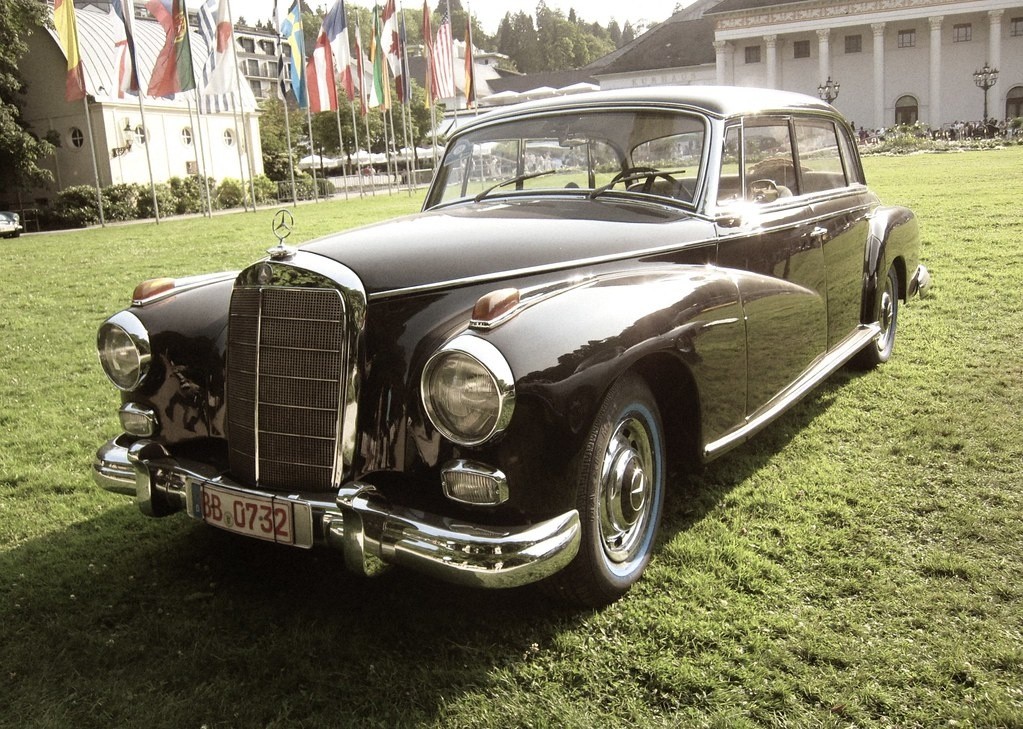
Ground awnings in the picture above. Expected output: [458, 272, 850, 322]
[194, 0, 235, 97]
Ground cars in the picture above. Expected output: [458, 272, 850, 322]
[90, 86, 926, 616]
[0, 211, 23, 238]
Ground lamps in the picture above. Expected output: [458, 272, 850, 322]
[111, 121, 137, 157]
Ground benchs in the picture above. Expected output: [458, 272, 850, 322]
[627, 172, 846, 203]
[719, 180, 793, 204]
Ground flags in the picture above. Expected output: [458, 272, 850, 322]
[322, 0, 355, 101]
[421, 0, 438, 110]
[353, 11, 368, 117]
[110, 0, 140, 100]
[52, 0, 85, 102]
[380, 0, 404, 104]
[144, 0, 195, 102]
[271, 0, 287, 101]
[433, 6, 456, 100]
[281, 4, 308, 109]
[306, 0, 339, 113]
[463, 16, 475, 106]
[369, 7, 384, 109]
[400, 15, 412, 104]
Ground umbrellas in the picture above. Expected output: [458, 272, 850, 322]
[298, 154, 336, 175]
[450, 141, 506, 157]
[480, 81, 601, 105]
[337, 149, 380, 169]
[378, 145, 445, 172]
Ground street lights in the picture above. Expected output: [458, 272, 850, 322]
[972, 61, 999, 138]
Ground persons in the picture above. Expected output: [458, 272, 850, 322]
[914, 123, 944, 140]
[523, 152, 565, 172]
[851, 121, 909, 146]
[354, 165, 377, 176]
[949, 116, 1022, 140]
